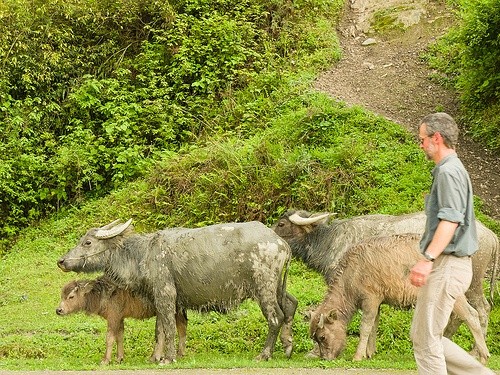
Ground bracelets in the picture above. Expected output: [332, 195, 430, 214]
[423, 251, 436, 262]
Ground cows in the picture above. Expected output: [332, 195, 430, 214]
[55, 218, 298, 365]
[270, 209, 500, 362]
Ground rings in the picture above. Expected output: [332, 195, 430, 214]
[418, 277, 423, 281]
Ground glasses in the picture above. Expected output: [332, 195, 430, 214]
[418, 133, 443, 144]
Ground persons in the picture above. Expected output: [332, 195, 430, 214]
[409, 112, 496, 375]
